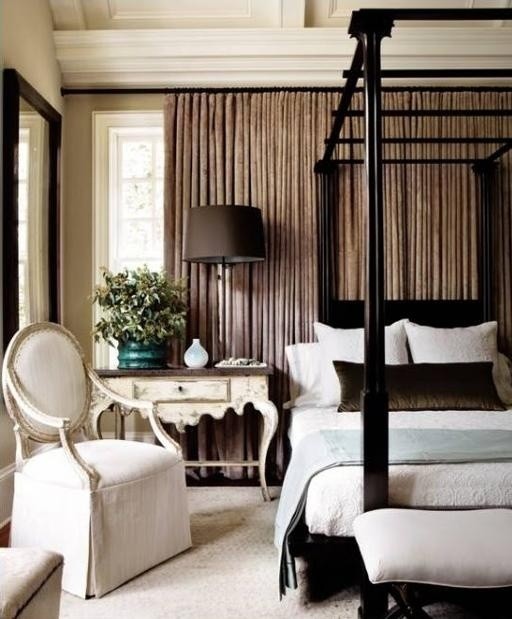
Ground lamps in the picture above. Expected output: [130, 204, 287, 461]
[182, 206, 266, 369]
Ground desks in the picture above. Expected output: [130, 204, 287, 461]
[82, 366, 280, 506]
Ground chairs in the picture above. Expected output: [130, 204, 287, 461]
[2, 321, 193, 600]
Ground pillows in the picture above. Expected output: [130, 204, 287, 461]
[332, 360, 507, 413]
[402, 319, 510, 407]
[314, 318, 409, 408]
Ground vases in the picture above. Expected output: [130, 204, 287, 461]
[87, 264, 191, 372]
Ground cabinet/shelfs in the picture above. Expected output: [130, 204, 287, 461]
[132, 377, 231, 404]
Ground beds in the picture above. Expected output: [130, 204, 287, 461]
[297, 7, 511, 614]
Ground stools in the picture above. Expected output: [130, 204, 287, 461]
[353, 506, 512, 619]
[1, 549, 63, 619]
